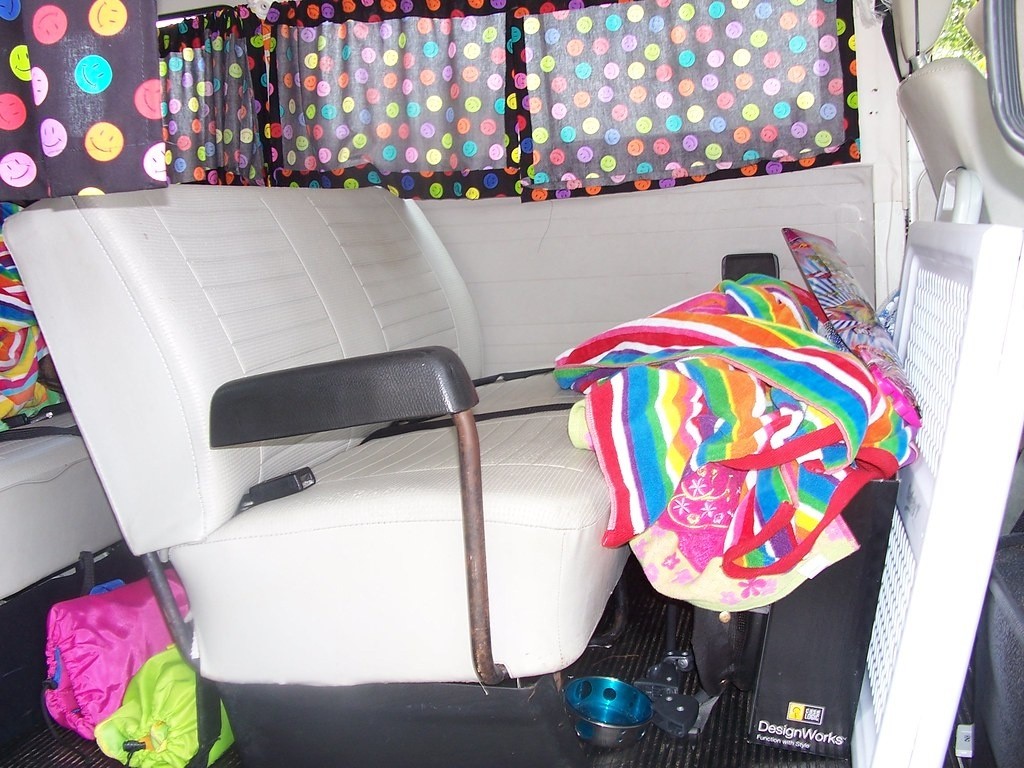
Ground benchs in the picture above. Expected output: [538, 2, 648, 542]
[0, 184, 632, 768]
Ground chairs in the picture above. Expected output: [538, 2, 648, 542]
[892, 0, 1024, 225]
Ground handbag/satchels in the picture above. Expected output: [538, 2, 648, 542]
[46, 569, 193, 739]
[93, 646, 234, 768]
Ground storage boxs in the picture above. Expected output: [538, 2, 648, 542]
[747, 477, 901, 761]
